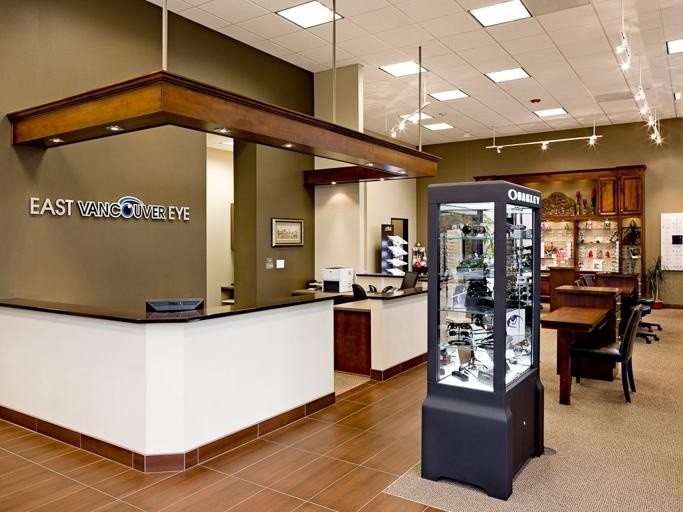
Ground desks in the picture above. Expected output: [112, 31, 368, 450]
[540, 273, 640, 405]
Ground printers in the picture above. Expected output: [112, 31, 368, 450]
[321, 266, 354, 293]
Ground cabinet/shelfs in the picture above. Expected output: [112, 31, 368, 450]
[420, 181, 544, 501]
[472, 164, 646, 304]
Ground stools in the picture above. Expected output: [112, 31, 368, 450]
[630, 295, 662, 344]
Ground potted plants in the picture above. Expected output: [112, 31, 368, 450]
[650, 256, 666, 309]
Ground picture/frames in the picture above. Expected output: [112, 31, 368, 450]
[270, 217, 304, 247]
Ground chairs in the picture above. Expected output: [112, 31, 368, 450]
[569, 303, 643, 404]
[349, 283, 367, 300]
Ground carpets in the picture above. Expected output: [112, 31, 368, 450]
[381, 306, 682, 511]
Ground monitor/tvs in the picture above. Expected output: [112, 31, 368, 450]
[400, 271, 419, 289]
[145, 298, 205, 313]
